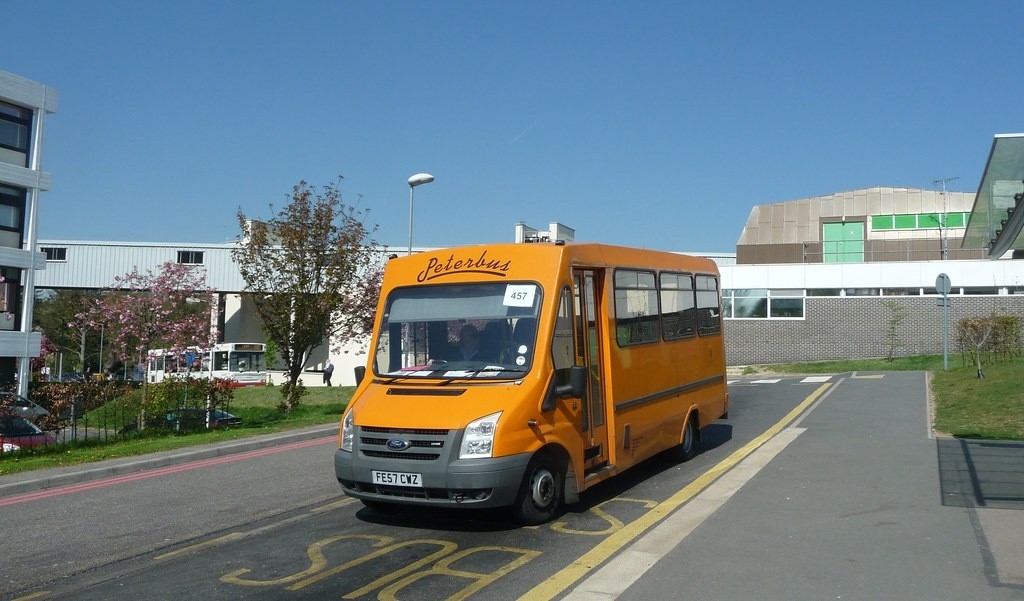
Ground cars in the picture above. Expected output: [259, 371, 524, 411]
[0, 391, 55, 431]
[116, 407, 242, 436]
[0, 415, 57, 455]
[57, 372, 84, 382]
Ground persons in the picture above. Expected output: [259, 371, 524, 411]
[324, 360, 334, 387]
[427, 324, 488, 366]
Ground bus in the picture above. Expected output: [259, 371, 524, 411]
[330, 241, 731, 528]
[147, 342, 267, 388]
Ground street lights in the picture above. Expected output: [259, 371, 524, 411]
[401, 172, 435, 369]
[185, 296, 215, 430]
[97, 322, 104, 373]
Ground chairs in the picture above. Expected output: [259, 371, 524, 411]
[631, 307, 719, 342]
[480, 317, 538, 364]
